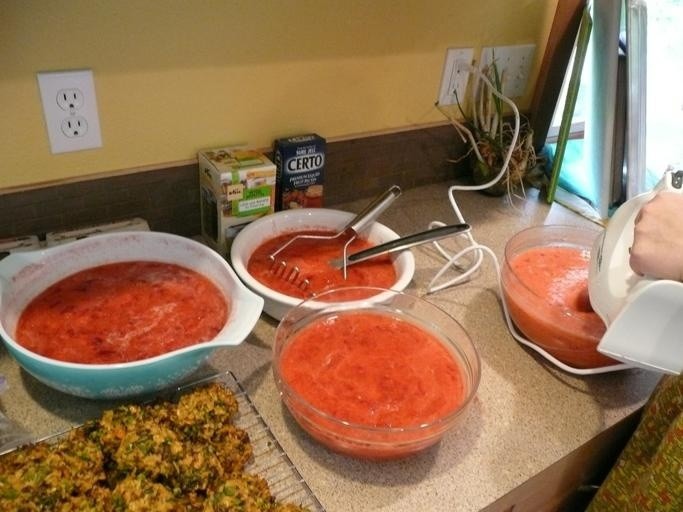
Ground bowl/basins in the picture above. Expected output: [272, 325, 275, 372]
[502, 223, 625, 378]
[0, 228, 265, 400]
[228, 207, 433, 321]
[271, 283, 481, 461]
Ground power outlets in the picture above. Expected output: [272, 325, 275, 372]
[36, 69, 103, 156]
[474, 45, 535, 100]
[439, 49, 476, 107]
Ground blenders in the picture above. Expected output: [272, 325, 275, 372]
[586, 164, 683, 378]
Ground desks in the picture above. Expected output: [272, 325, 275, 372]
[1, 169, 672, 510]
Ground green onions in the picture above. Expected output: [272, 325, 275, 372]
[435, 47, 506, 176]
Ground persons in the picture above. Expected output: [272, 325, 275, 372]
[581, 191, 683, 512]
